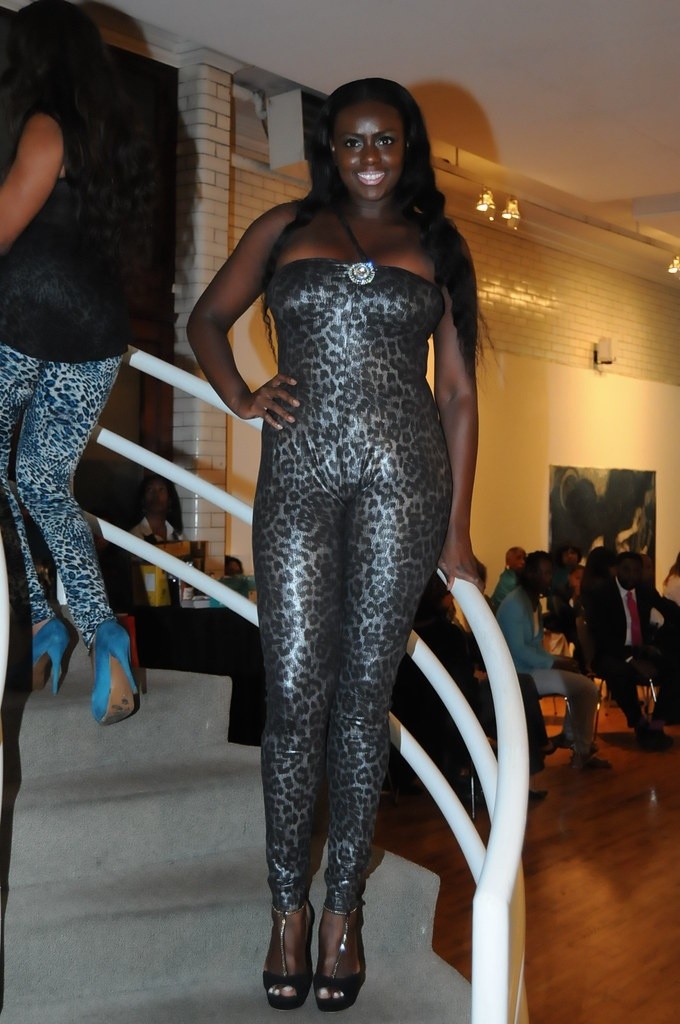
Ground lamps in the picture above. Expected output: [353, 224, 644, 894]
[668, 256, 680, 274]
[501, 195, 521, 219]
[474, 186, 495, 221]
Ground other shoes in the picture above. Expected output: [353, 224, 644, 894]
[529, 789, 548, 801]
[634, 726, 674, 752]
[584, 756, 613, 769]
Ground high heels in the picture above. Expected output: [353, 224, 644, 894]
[312, 912, 366, 1013]
[32, 617, 71, 698]
[90, 620, 139, 725]
[263, 901, 315, 1010]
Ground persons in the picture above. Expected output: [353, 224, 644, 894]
[126, 472, 186, 594]
[185, 78, 487, 1011]
[225, 556, 244, 576]
[392, 544, 680, 807]
[0, 2, 148, 726]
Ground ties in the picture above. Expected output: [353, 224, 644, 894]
[625, 591, 642, 646]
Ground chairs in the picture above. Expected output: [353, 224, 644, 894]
[447, 652, 662, 821]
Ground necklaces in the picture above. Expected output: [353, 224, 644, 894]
[333, 202, 404, 288]
